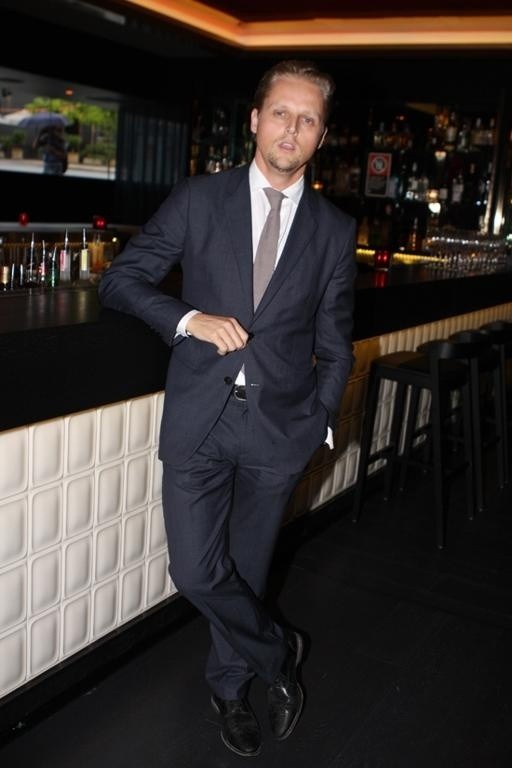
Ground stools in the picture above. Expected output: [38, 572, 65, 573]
[353, 318, 512, 552]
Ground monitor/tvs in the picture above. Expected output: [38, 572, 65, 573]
[0, 66, 122, 180]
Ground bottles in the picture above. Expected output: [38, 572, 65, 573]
[1, 227, 104, 292]
[207, 144, 228, 175]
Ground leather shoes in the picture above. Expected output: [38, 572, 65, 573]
[262, 628, 308, 743]
[209, 688, 266, 758]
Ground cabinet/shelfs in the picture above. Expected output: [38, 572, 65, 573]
[198, 103, 512, 258]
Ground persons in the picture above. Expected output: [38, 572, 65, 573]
[93, 54, 364, 762]
[32, 130, 68, 175]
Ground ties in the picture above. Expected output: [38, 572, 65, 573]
[239, 186, 285, 376]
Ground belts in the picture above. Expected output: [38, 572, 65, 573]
[228, 382, 248, 402]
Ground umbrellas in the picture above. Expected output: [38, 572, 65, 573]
[0, 106, 68, 131]
